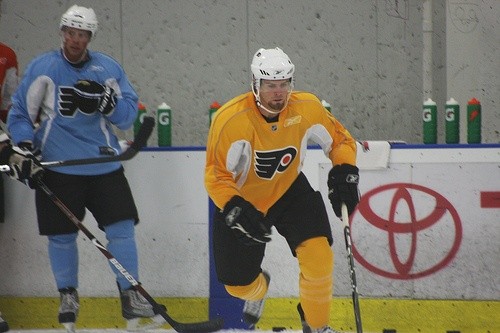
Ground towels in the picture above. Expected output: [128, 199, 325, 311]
[357, 141, 390, 171]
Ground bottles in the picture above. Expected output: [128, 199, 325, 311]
[466, 98, 482, 145]
[422, 98, 438, 144]
[133, 103, 146, 133]
[157, 102, 172, 149]
[208, 102, 220, 127]
[322, 100, 331, 113]
[443, 97, 462, 144]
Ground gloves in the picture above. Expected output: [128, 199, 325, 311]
[0, 146, 44, 191]
[222, 195, 272, 243]
[13, 140, 42, 161]
[327, 163, 360, 219]
[73, 80, 117, 116]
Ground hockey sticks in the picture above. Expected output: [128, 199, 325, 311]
[0, 116, 156, 172]
[38, 176, 222, 333]
[341, 200, 362, 333]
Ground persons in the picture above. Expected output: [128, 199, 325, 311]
[0, 43, 18, 333]
[6, 4, 168, 324]
[204, 46, 361, 333]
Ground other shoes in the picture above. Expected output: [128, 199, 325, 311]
[0, 312, 9, 332]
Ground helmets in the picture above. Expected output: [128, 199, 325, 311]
[59, 3, 97, 64]
[251, 46, 295, 113]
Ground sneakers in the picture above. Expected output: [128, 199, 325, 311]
[297, 302, 342, 333]
[121, 288, 167, 331]
[58, 287, 79, 333]
[241, 271, 271, 328]
